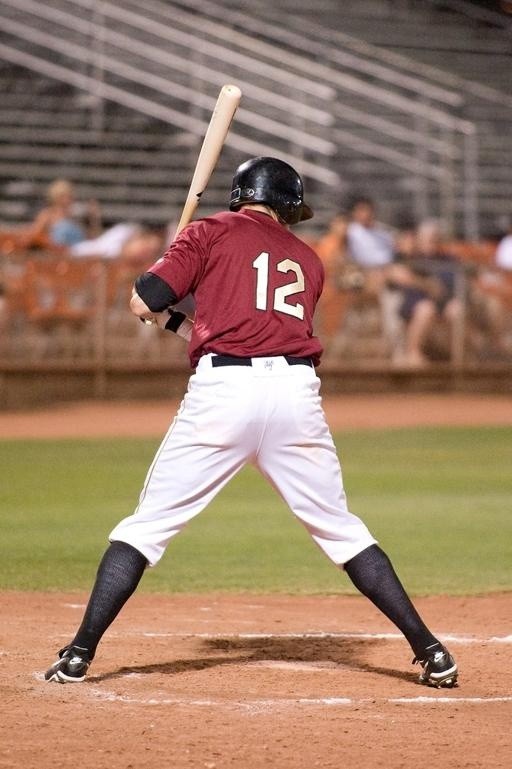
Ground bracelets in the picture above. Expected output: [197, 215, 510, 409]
[164, 311, 187, 334]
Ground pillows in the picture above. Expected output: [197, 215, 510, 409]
[228, 156, 314, 225]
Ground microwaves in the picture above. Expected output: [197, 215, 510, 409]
[140, 84, 242, 324]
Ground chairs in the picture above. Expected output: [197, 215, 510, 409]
[410, 647, 457, 690]
[34, 645, 94, 684]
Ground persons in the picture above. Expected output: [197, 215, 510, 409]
[344, 195, 403, 334]
[46, 156, 459, 689]
[387, 214, 467, 364]
[32, 177, 86, 251]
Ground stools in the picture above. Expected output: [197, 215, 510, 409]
[206, 349, 316, 372]
